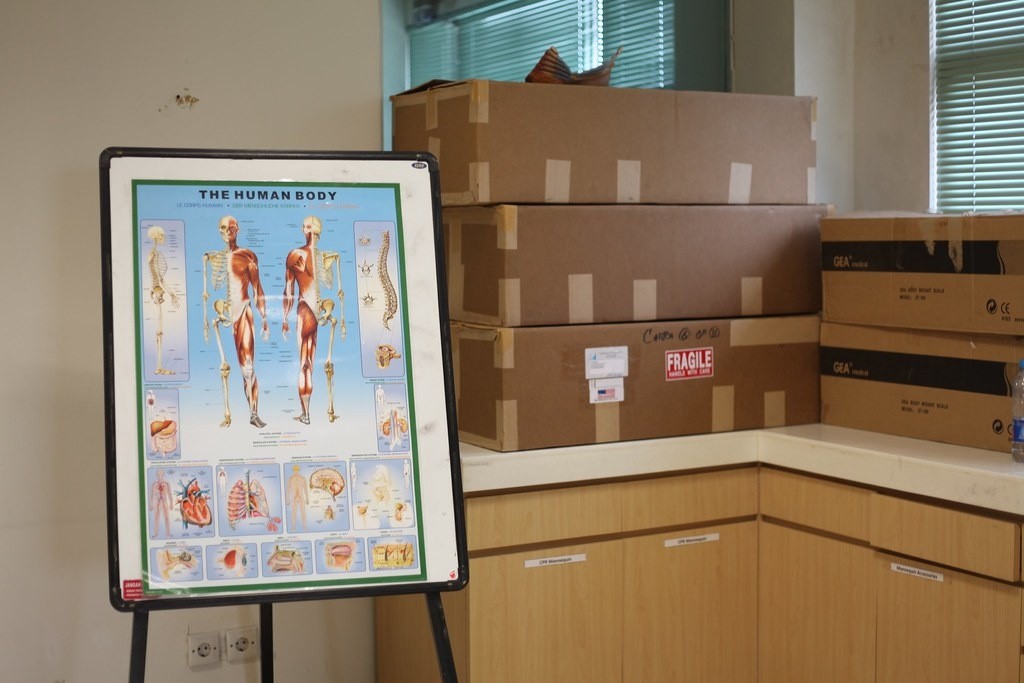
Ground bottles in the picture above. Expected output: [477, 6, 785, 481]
[1012, 359, 1024, 463]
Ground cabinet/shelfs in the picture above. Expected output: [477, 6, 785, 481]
[374, 425, 1024, 683]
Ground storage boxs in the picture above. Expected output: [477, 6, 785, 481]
[389, 77, 1023, 454]
